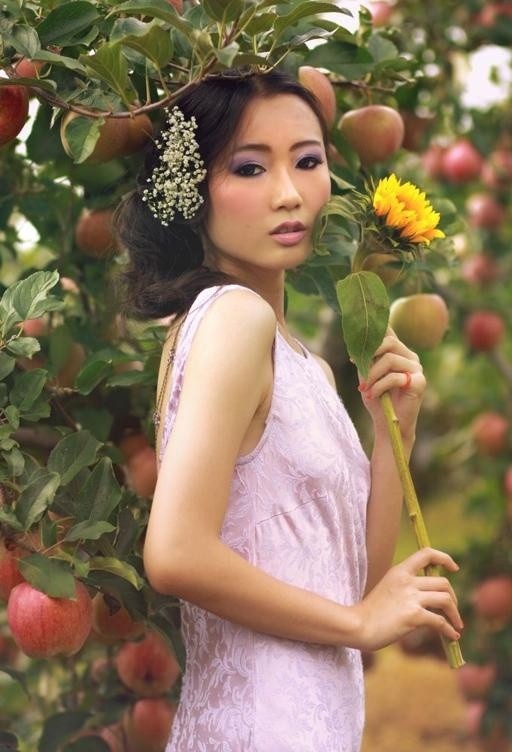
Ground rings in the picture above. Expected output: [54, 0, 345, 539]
[403, 369, 413, 388]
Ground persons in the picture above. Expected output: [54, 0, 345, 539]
[109, 68, 464, 751]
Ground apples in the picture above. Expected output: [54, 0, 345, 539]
[467, 701, 511, 749]
[470, 412, 507, 456]
[15, 276, 127, 386]
[46, 510, 71, 548]
[116, 631, 177, 696]
[89, 592, 146, 644]
[367, 253, 401, 285]
[459, 663, 497, 698]
[76, 207, 117, 258]
[473, 576, 510, 620]
[123, 699, 175, 752]
[60, 107, 129, 161]
[122, 104, 152, 154]
[389, 292, 449, 351]
[2, 86, 28, 145]
[423, 0, 512, 353]
[10, 580, 92, 658]
[1, 535, 39, 604]
[119, 434, 151, 463]
[296, 66, 336, 133]
[124, 450, 157, 497]
[15, 47, 59, 77]
[338, 104, 405, 161]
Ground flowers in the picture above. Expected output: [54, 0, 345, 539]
[306, 171, 467, 669]
